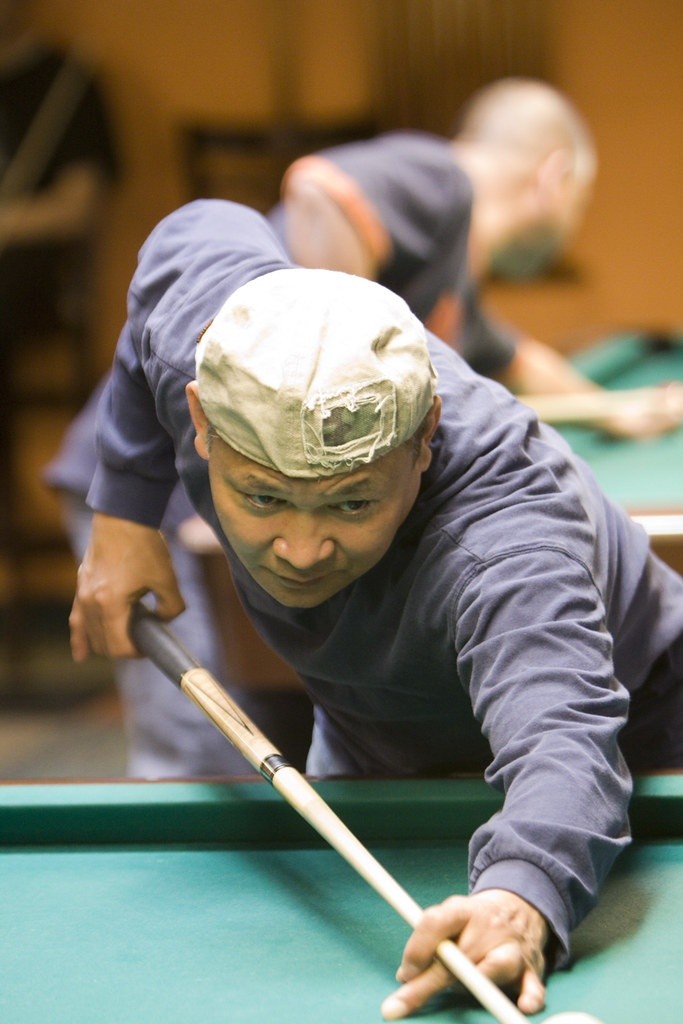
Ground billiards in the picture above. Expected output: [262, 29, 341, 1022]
[540, 1012, 603, 1024]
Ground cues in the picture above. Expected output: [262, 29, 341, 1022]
[508, 385, 666, 425]
[125, 601, 538, 1024]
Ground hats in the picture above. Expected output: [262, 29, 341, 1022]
[196, 268, 436, 480]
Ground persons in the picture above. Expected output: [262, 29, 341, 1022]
[0, 0, 128, 701]
[38, 78, 683, 781]
[68, 200, 683, 1021]
